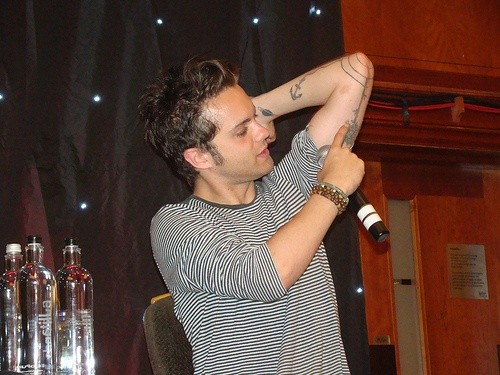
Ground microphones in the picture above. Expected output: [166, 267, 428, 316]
[316, 145, 390, 242]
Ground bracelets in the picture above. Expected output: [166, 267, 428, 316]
[318, 181, 349, 204]
[311, 184, 347, 215]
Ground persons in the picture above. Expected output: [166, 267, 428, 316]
[139, 51, 374, 375]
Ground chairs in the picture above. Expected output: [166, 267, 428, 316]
[142, 298, 194, 375]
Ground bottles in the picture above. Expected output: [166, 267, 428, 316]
[19, 235, 57, 375]
[0, 244, 23, 372]
[54, 238, 94, 375]
